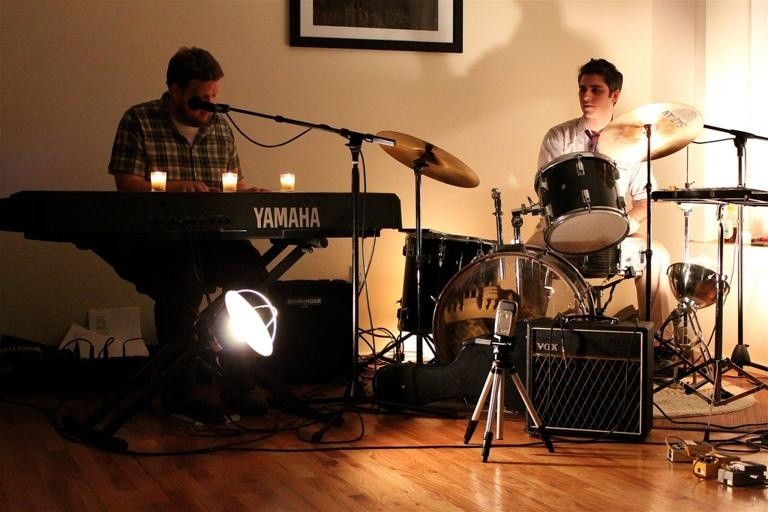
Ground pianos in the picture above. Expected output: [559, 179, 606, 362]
[0, 190, 400, 240]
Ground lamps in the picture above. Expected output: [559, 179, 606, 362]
[199, 287, 281, 371]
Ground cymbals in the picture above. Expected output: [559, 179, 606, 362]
[596, 103, 705, 163]
[372, 128, 478, 188]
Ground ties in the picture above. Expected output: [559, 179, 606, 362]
[586, 130, 601, 152]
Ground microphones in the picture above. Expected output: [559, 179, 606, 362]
[188, 96, 229, 113]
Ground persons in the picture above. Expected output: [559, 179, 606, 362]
[522, 58, 691, 378]
[109, 46, 269, 424]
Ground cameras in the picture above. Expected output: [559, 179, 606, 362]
[494, 298, 519, 337]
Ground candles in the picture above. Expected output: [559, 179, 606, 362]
[151, 171, 166, 193]
[222, 170, 237, 193]
[280, 173, 295, 192]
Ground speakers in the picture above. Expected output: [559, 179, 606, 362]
[238, 279, 353, 386]
[527, 325, 650, 443]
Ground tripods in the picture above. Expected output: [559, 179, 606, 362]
[653, 204, 768, 441]
[359, 160, 472, 411]
[227, 105, 460, 444]
[687, 149, 768, 394]
[463, 347, 555, 462]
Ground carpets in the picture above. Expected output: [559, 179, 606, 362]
[651, 374, 755, 419]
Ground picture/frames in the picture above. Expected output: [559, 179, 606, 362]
[290, 0, 463, 54]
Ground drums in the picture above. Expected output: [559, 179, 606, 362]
[432, 244, 594, 371]
[534, 152, 628, 259]
[558, 237, 643, 280]
[668, 261, 729, 308]
[396, 227, 505, 330]
